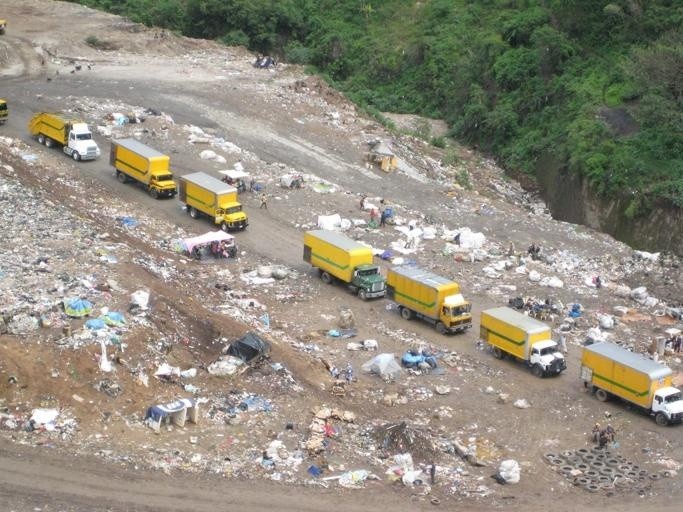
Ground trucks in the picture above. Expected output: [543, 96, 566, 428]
[580, 341, 683, 426]
[109, 138, 178, 198]
[479, 306, 567, 378]
[302, 229, 386, 301]
[178, 171, 248, 232]
[384, 265, 472, 334]
[27, 113, 101, 161]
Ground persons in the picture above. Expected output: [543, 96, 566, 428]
[569, 299, 583, 317]
[379, 211, 385, 226]
[220, 175, 256, 194]
[508, 241, 514, 256]
[596, 275, 602, 287]
[673, 334, 681, 353]
[360, 197, 365, 211]
[527, 241, 535, 259]
[592, 275, 601, 288]
[510, 293, 559, 321]
[536, 243, 545, 259]
[233, 159, 243, 172]
[590, 420, 616, 451]
[259, 193, 267, 210]
[403, 225, 414, 248]
[370, 208, 375, 222]
